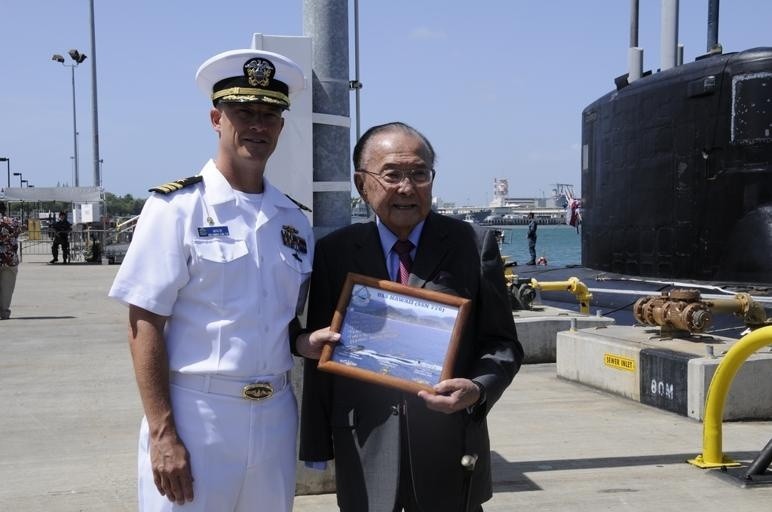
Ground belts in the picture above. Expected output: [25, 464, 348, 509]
[172, 373, 286, 401]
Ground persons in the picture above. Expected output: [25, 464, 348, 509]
[298, 121, 523, 512]
[526, 211, 539, 266]
[107, 48, 342, 511]
[48, 209, 72, 264]
[0, 201, 19, 322]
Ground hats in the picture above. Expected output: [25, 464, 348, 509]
[196, 48, 305, 111]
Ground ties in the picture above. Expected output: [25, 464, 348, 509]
[393, 243, 415, 286]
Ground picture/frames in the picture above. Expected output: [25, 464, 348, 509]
[314, 266, 477, 400]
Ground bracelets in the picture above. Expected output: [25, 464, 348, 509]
[471, 381, 485, 414]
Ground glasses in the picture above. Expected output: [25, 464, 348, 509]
[362, 165, 436, 185]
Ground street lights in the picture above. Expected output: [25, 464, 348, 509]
[0, 158, 11, 218]
[28, 185, 36, 219]
[52, 50, 87, 226]
[22, 180, 29, 229]
[14, 173, 23, 226]
